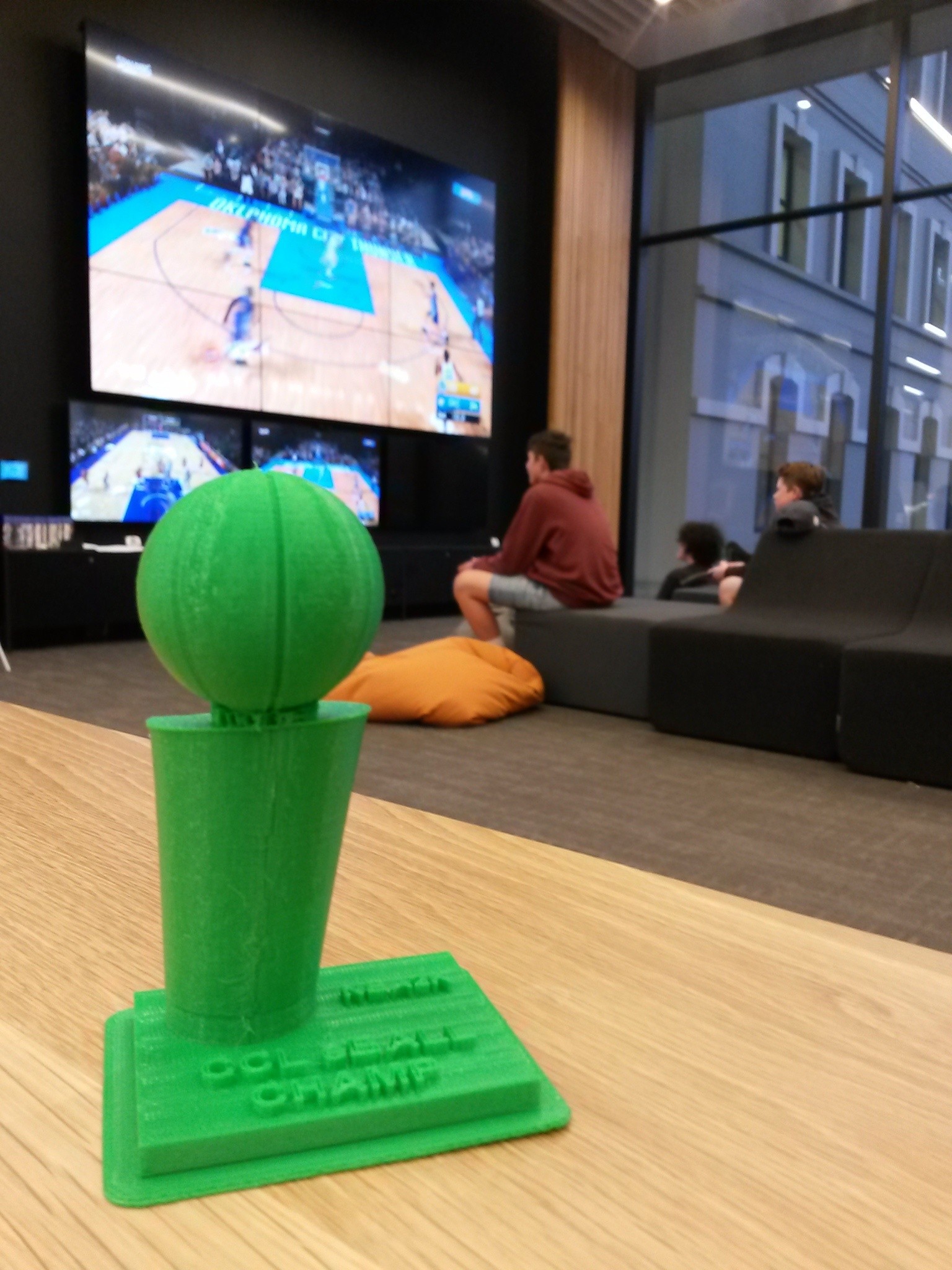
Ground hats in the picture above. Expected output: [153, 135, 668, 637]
[774, 500, 822, 533]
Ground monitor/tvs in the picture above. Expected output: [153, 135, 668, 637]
[78, 20, 499, 447]
[63, 396, 390, 538]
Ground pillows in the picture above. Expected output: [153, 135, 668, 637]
[323, 635, 541, 724]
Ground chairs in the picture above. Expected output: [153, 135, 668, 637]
[836, 531, 952, 790]
[648, 525, 941, 762]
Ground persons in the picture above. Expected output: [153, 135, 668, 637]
[453, 430, 623, 649]
[226, 213, 256, 271]
[70, 403, 381, 525]
[656, 521, 725, 600]
[422, 279, 445, 339]
[87, 106, 494, 293]
[433, 351, 466, 420]
[708, 463, 833, 607]
[224, 288, 257, 363]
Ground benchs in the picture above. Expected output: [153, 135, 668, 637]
[512, 586, 730, 720]
